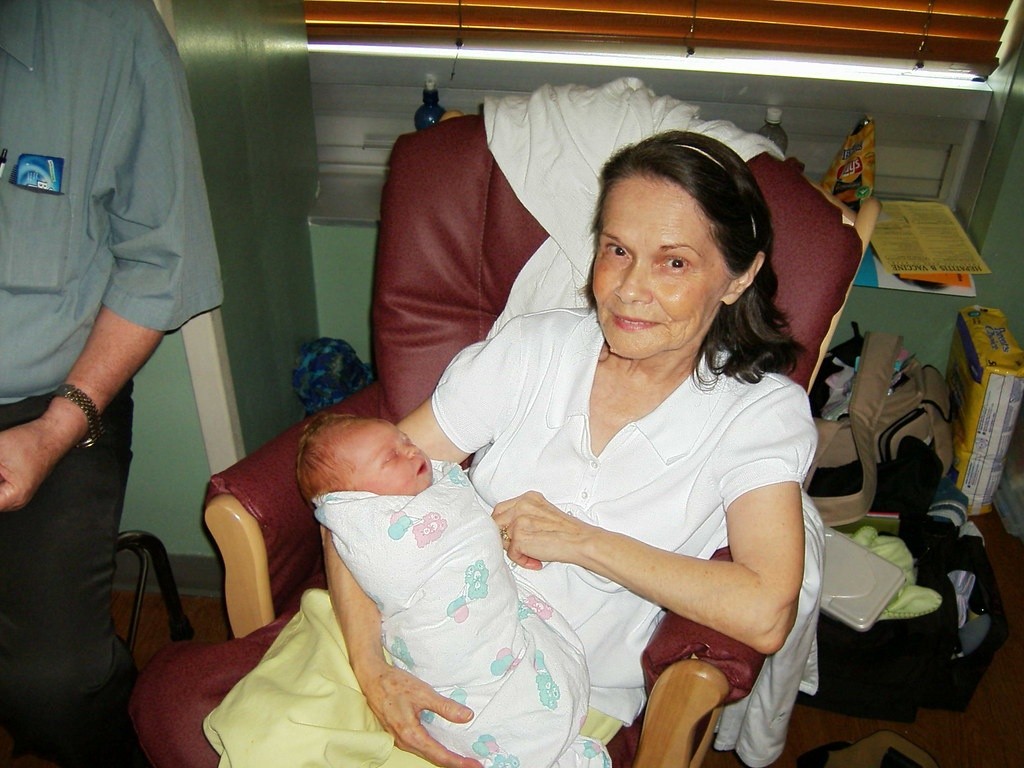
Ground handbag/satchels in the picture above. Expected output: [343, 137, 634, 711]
[804, 332, 954, 529]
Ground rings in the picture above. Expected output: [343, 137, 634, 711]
[499, 524, 512, 542]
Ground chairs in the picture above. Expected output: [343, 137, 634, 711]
[114, 530, 195, 653]
[128, 110, 881, 768]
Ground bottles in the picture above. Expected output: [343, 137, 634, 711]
[758, 107, 787, 158]
[414, 89, 446, 131]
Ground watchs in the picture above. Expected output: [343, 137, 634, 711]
[53, 383, 108, 451]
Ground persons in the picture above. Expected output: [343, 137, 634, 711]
[0, 0, 226, 768]
[294, 407, 611, 768]
[201, 128, 822, 768]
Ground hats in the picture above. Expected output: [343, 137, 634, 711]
[293, 337, 372, 420]
